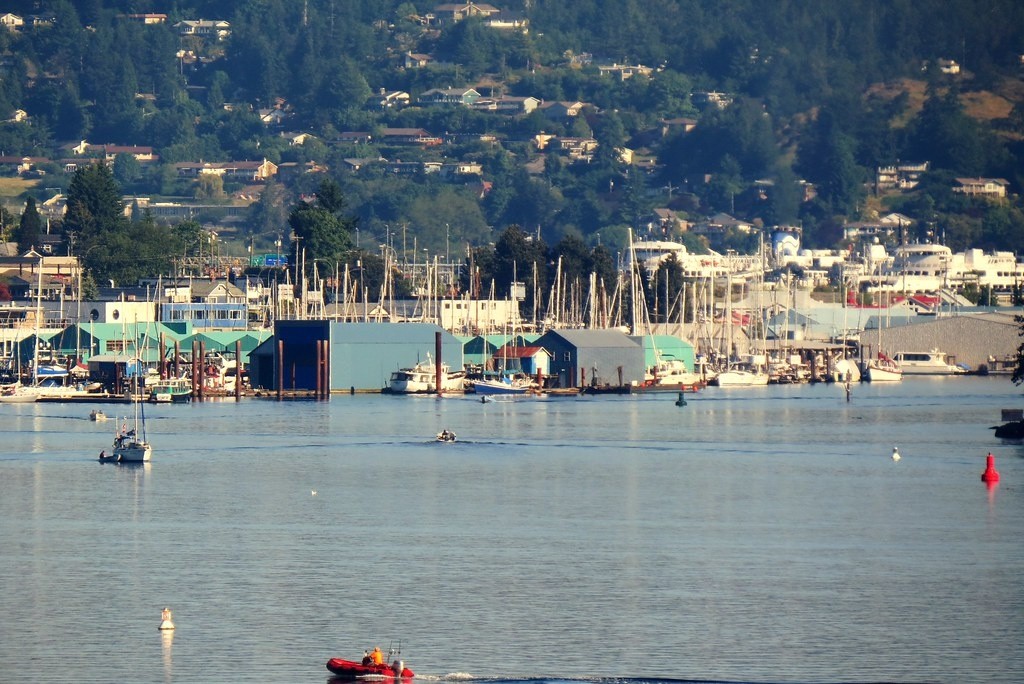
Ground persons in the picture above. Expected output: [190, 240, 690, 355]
[370, 647, 383, 665]
[161, 607, 171, 620]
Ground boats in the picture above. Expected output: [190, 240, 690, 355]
[436, 430, 458, 443]
[327, 657, 415, 684]
[389, 362, 449, 392]
[91, 413, 105, 421]
[100, 454, 123, 463]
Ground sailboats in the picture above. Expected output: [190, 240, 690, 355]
[0, 256, 193, 405]
[463, 295, 530, 395]
[628, 226, 967, 385]
[111, 314, 152, 463]
